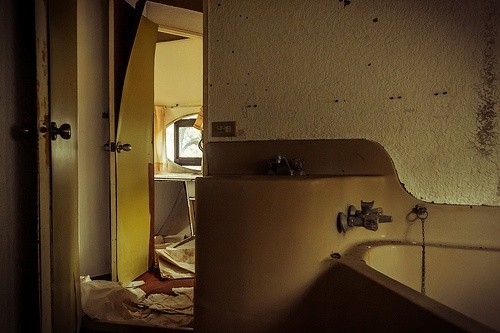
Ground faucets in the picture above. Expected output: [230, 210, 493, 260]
[339, 201, 393, 232]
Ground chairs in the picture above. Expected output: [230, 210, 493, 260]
[186, 180, 195, 232]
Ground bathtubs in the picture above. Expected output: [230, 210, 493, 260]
[332, 240, 500, 333]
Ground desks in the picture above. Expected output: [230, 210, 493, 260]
[155, 172, 202, 182]
